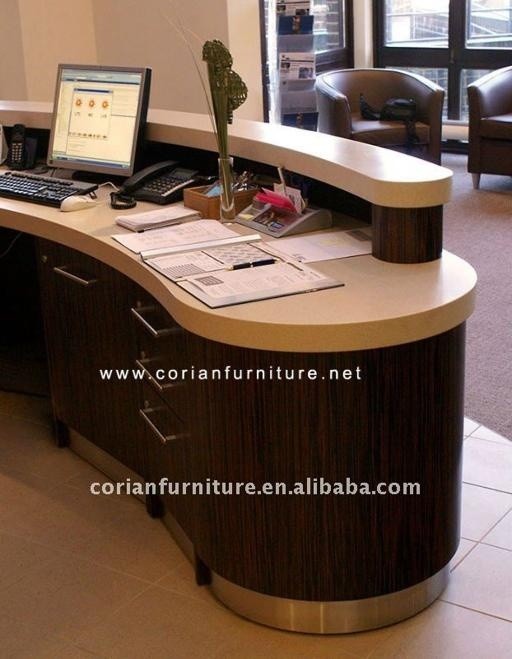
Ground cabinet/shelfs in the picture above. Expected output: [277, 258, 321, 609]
[33, 236, 212, 587]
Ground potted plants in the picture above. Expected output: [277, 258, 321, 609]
[159, 9, 248, 224]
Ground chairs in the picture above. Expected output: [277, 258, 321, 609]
[313, 68, 445, 166]
[466, 65, 512, 189]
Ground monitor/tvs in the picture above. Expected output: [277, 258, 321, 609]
[46, 63, 152, 185]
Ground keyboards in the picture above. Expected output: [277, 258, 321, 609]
[0, 170, 98, 208]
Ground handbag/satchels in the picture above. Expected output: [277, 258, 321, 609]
[382, 98, 416, 122]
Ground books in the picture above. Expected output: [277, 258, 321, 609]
[143, 241, 345, 309]
[112, 219, 240, 254]
[277, 16, 295, 35]
[299, 15, 314, 34]
[116, 204, 203, 233]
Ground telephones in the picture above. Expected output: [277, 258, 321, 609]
[7, 124, 27, 170]
[119, 160, 201, 204]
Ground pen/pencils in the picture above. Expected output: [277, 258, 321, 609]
[227, 257, 280, 271]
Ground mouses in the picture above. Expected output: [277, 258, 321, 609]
[60, 195, 96, 211]
